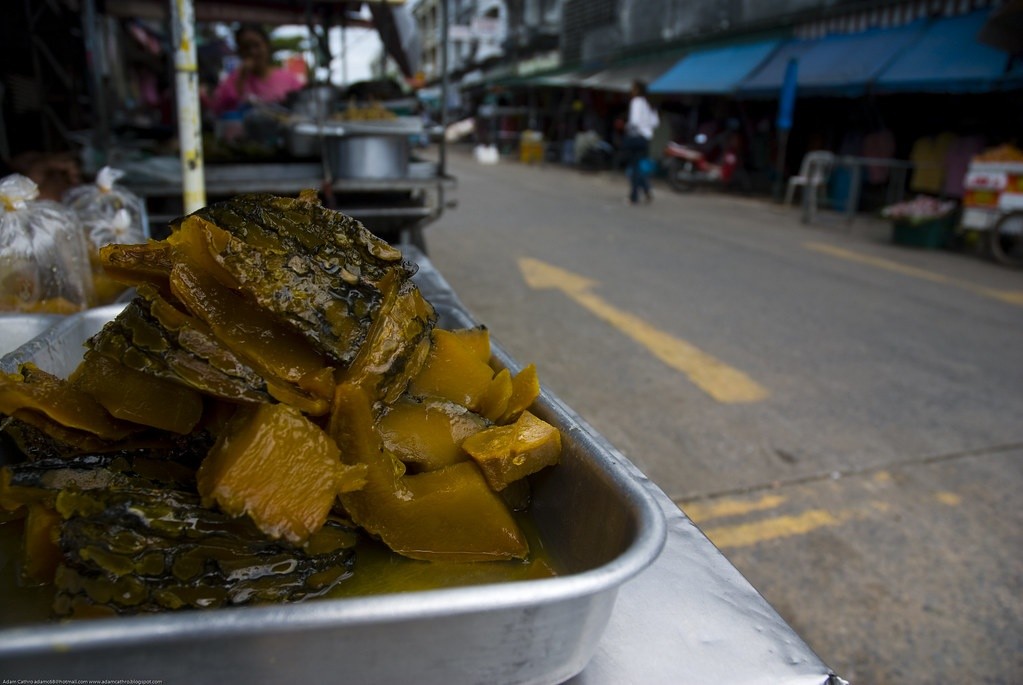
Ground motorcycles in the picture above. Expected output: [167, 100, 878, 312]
[662, 134, 765, 194]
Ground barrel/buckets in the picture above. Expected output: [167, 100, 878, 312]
[324, 133, 408, 181]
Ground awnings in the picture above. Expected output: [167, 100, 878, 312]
[646, 10, 1023, 93]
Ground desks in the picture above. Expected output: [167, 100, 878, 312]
[804, 154, 917, 232]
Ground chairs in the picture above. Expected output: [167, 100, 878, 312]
[785, 149, 835, 215]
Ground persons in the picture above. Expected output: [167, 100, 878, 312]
[212, 23, 309, 118]
[624, 79, 660, 205]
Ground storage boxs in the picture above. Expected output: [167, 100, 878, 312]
[888, 213, 955, 250]
